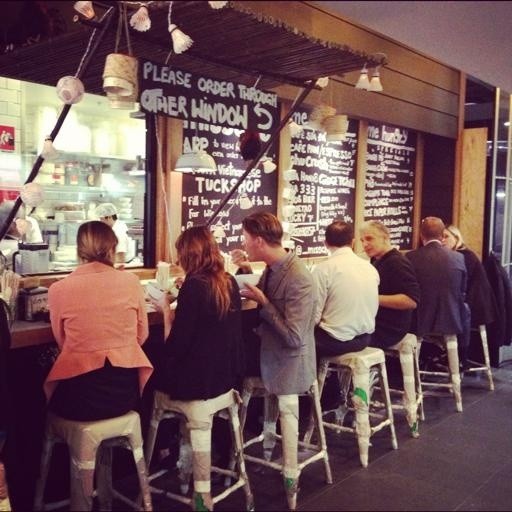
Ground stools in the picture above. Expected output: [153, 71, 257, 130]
[352, 333, 427, 439]
[136, 390, 254, 512]
[35, 411, 152, 512]
[301, 346, 398, 467]
[416, 333, 463, 412]
[225, 378, 333, 510]
[461, 325, 494, 392]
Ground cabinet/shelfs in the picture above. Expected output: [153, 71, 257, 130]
[41, 150, 137, 198]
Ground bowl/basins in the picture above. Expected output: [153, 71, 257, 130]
[234, 275, 259, 289]
[146, 282, 175, 301]
[117, 198, 134, 219]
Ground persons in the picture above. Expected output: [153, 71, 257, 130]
[41, 218, 156, 422]
[151, 224, 243, 467]
[0, 298, 44, 449]
[358, 221, 422, 349]
[231, 212, 319, 398]
[404, 214, 471, 337]
[94, 203, 130, 261]
[313, 220, 382, 358]
[440, 225, 502, 327]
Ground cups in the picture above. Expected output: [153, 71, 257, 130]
[12, 242, 50, 274]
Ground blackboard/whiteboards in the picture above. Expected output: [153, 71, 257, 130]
[356, 118, 423, 256]
[137, 60, 285, 134]
[281, 102, 358, 264]
[143, 113, 278, 271]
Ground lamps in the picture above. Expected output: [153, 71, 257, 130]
[172, 148, 218, 173]
